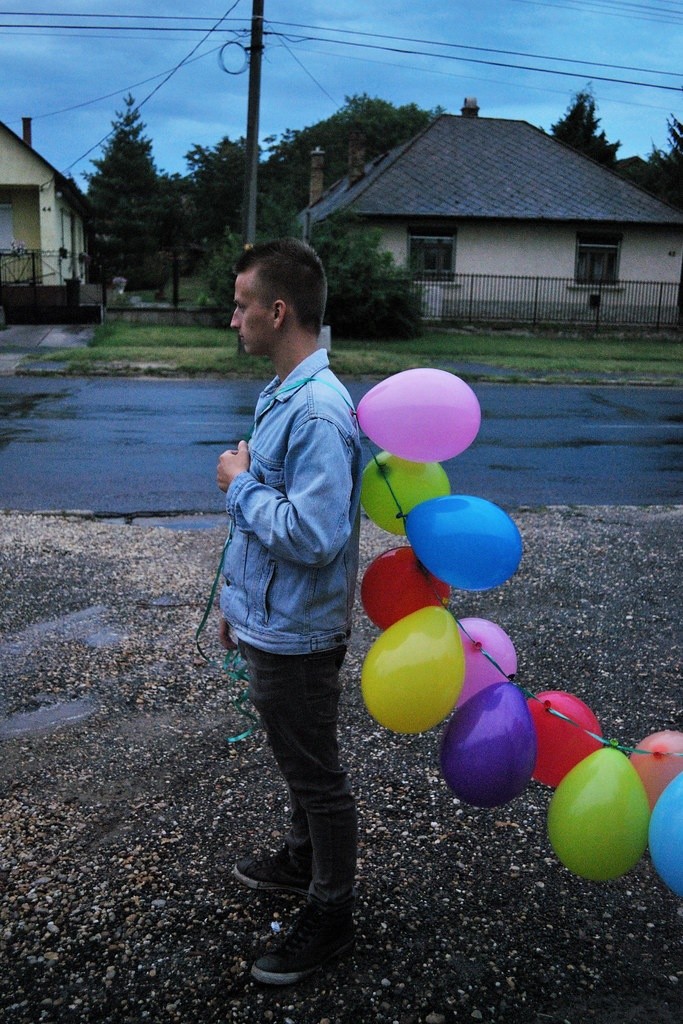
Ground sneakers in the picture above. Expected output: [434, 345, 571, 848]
[253, 900, 355, 985]
[233, 844, 312, 895]
